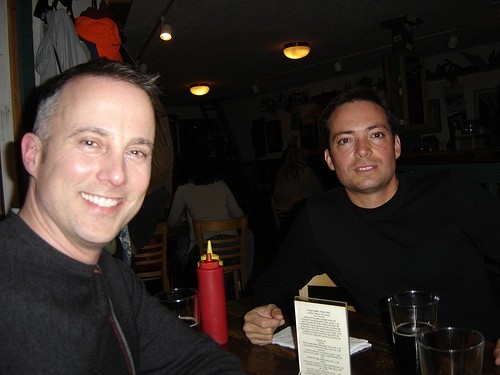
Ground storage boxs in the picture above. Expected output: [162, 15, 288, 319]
[456, 134, 486, 150]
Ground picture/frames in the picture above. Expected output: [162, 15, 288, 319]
[474, 88, 500, 126]
[421, 98, 441, 134]
[300, 117, 318, 150]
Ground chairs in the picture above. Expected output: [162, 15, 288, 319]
[193, 217, 247, 299]
[271, 197, 301, 248]
[132, 223, 168, 291]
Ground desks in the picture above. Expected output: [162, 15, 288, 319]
[177, 291, 500, 375]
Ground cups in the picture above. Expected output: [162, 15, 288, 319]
[166, 287, 193, 326]
[416, 327, 485, 375]
[388, 291, 437, 375]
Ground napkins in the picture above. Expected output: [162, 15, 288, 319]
[272, 325, 372, 356]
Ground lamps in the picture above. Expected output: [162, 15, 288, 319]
[252, 81, 261, 95]
[447, 32, 458, 48]
[283, 42, 310, 59]
[333, 59, 343, 72]
[190, 84, 210, 96]
[159, 15, 172, 41]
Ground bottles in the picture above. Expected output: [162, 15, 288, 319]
[196, 253, 229, 348]
[197, 240, 223, 268]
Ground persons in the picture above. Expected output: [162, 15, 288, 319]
[242, 85, 500, 367]
[0, 55, 247, 375]
